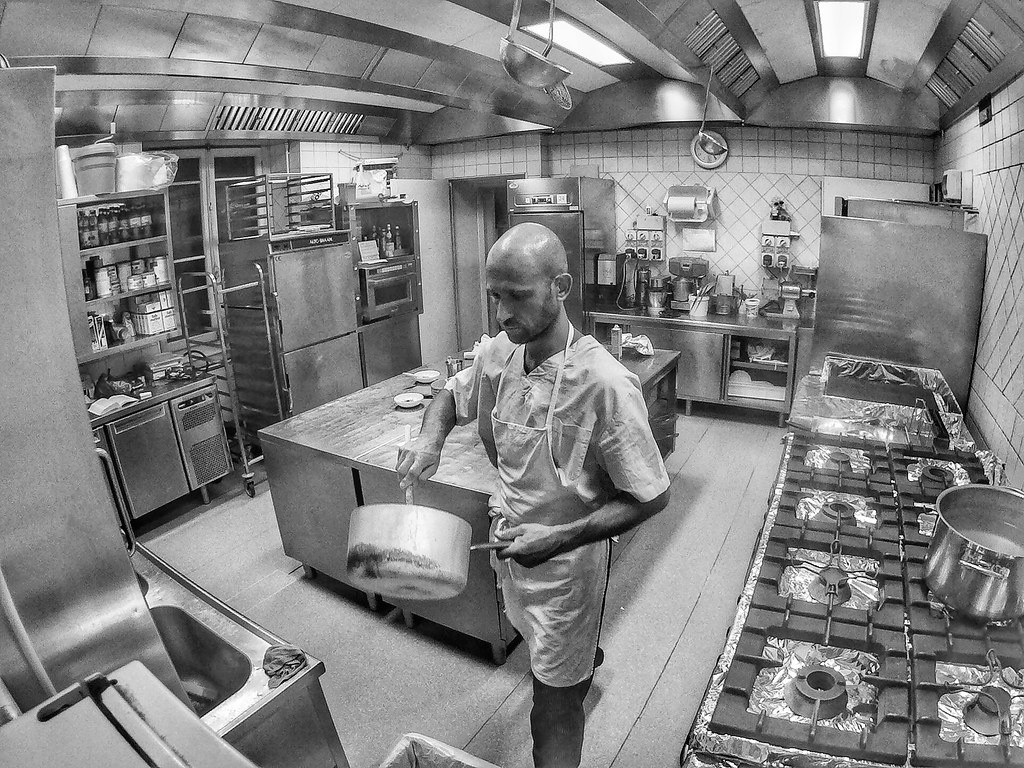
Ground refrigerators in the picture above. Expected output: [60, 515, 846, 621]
[219, 234, 364, 439]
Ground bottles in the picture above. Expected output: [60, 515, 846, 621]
[77, 203, 153, 248]
[395, 225, 401, 250]
[363, 221, 395, 257]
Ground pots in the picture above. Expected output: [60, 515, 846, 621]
[920, 484, 1024, 618]
[342, 505, 518, 600]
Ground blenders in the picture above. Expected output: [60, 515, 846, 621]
[716, 274, 734, 315]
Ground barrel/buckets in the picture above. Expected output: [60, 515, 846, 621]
[70, 142, 116, 195]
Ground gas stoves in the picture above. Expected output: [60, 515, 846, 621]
[713, 425, 1024, 767]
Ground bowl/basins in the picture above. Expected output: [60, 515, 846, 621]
[394, 392, 424, 408]
[413, 370, 440, 383]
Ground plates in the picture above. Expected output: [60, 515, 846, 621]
[407, 384, 432, 397]
[431, 379, 446, 390]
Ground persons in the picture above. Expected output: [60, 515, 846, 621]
[397, 222, 670, 768]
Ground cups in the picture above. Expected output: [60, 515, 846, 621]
[745, 298, 760, 318]
[689, 295, 709, 317]
[399, 193, 406, 199]
[445, 356, 463, 376]
[57, 144, 80, 199]
[94, 256, 168, 298]
[338, 183, 357, 205]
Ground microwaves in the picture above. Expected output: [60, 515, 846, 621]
[358, 263, 420, 321]
